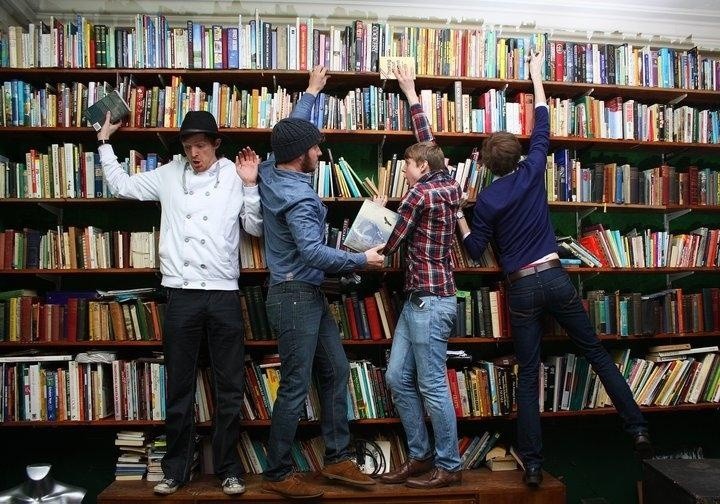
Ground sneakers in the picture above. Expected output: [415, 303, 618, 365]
[153, 476, 180, 494]
[222, 475, 247, 495]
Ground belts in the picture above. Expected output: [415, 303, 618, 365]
[405, 291, 431, 309]
[503, 259, 562, 285]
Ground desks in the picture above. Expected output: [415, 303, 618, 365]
[96, 467, 568, 504]
[636, 459, 720, 503]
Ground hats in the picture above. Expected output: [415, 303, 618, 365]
[270, 117, 322, 164]
[177, 110, 219, 139]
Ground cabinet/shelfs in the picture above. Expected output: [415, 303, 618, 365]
[0, 65, 719, 429]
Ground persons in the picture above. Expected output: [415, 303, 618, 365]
[260, 66, 386, 499]
[374, 62, 464, 488]
[99, 111, 263, 496]
[456, 50, 652, 485]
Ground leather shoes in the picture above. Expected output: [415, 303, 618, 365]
[405, 466, 463, 490]
[320, 460, 377, 486]
[261, 475, 324, 498]
[380, 458, 434, 484]
[633, 433, 654, 460]
[521, 467, 544, 485]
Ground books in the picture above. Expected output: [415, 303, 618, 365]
[3, 22, 720, 474]
